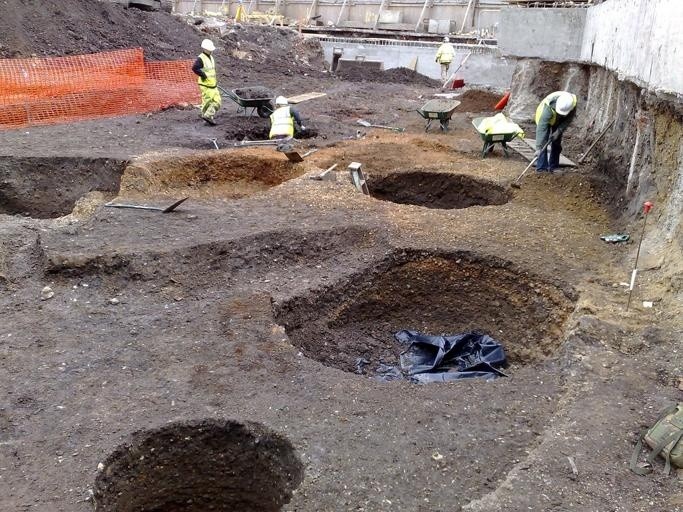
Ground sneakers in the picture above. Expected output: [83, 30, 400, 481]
[198, 113, 217, 125]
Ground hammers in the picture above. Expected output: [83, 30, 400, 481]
[310, 163, 338, 180]
[578, 120, 615, 164]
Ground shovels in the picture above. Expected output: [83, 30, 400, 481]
[511, 138, 553, 189]
[284, 148, 317, 163]
[357, 118, 405, 132]
[237, 135, 293, 143]
[104, 196, 189, 213]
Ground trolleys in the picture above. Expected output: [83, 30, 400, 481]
[215, 84, 277, 122]
[415, 99, 461, 132]
[471, 114, 523, 160]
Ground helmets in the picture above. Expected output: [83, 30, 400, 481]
[201, 39, 216, 51]
[276, 96, 288, 105]
[443, 37, 449, 42]
[555, 92, 577, 118]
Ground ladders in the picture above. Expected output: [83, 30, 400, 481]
[348, 161, 371, 196]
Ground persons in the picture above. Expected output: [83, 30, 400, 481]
[434, 36, 456, 82]
[191, 38, 222, 126]
[534, 91, 578, 177]
[267, 95, 306, 140]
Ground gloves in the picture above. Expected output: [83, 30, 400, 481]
[552, 132, 560, 140]
[301, 125, 306, 130]
[534, 150, 541, 160]
